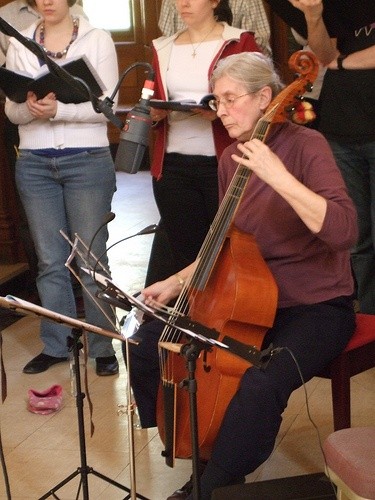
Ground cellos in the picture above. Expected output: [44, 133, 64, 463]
[154, 49, 321, 469]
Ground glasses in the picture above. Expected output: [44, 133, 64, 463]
[209, 91, 257, 111]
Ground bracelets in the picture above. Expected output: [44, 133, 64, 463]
[176, 274, 185, 289]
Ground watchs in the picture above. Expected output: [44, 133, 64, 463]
[337, 54, 348, 70]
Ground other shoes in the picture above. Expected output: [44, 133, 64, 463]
[95, 355, 120, 375]
[166, 477, 244, 500]
[23, 352, 70, 375]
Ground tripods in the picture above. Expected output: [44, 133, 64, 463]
[0, 295, 151, 500]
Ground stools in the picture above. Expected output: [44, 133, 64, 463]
[323, 426, 375, 500]
[315, 314, 375, 432]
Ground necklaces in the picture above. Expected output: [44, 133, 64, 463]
[189, 22, 218, 57]
[40, 18, 78, 58]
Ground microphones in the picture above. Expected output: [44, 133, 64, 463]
[86, 212, 115, 276]
[93, 225, 158, 289]
[113, 71, 156, 174]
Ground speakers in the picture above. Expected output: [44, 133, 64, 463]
[210, 471, 339, 500]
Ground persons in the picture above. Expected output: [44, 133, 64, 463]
[5, 0, 120, 376]
[288, 0, 375, 316]
[159, 0, 272, 58]
[0, 0, 88, 68]
[150, 0, 262, 289]
[122, 53, 359, 500]
